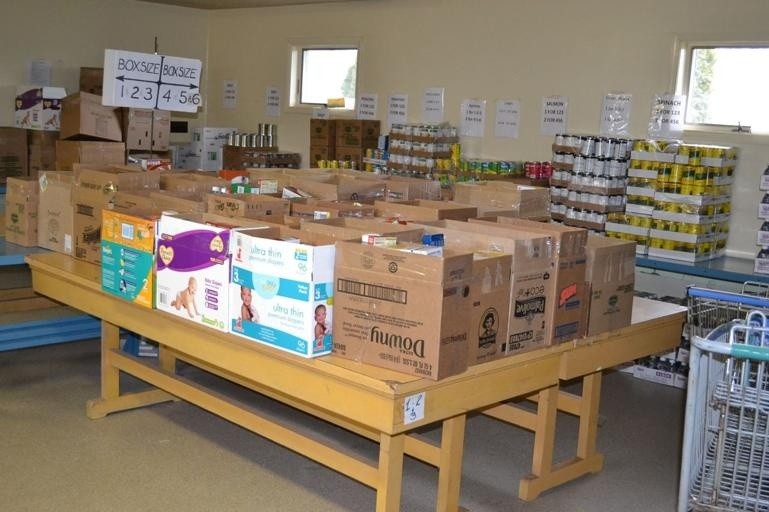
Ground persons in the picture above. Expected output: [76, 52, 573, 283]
[314, 304, 328, 340]
[480, 312, 497, 339]
[20, 110, 32, 127]
[44, 113, 58, 128]
[169, 277, 201, 319]
[239, 285, 257, 322]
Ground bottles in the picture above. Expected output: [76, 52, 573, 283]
[757, 162, 769, 258]
[551, 130, 635, 238]
[630, 293, 724, 379]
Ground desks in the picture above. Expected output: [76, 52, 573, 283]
[25, 248, 688, 512]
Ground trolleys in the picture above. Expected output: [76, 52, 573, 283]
[677, 279, 767, 512]
